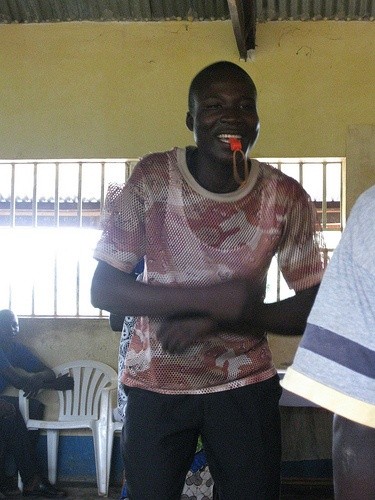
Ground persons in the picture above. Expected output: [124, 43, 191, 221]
[91, 60, 323, 500]
[279, 186, 375, 500]
[0, 310, 74, 496]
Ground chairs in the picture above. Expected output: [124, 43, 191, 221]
[19, 360, 124, 498]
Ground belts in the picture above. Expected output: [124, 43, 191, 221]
[23, 475, 68, 498]
[0, 476, 21, 495]
